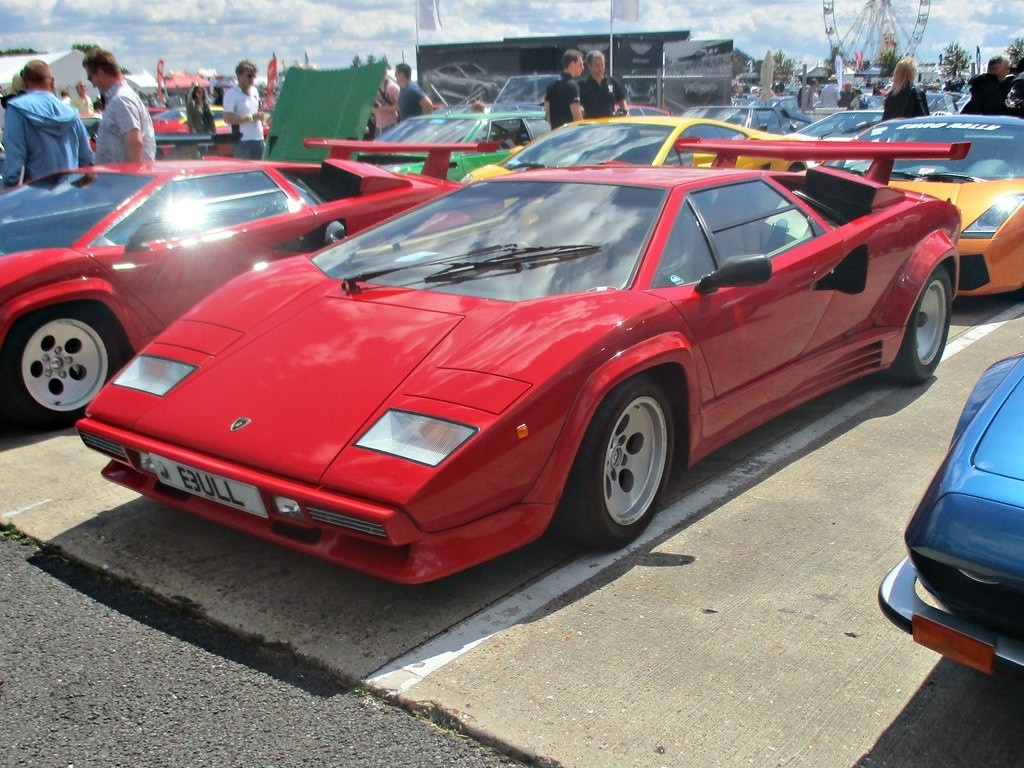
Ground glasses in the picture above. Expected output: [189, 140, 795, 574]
[244, 74, 256, 79]
[88, 70, 97, 81]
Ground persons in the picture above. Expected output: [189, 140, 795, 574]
[82, 48, 156, 166]
[373, 70, 400, 139]
[70, 81, 94, 117]
[544, 49, 584, 131]
[960, 56, 1009, 115]
[395, 63, 433, 123]
[882, 56, 930, 121]
[2, 60, 96, 190]
[93, 81, 281, 117]
[222, 59, 265, 160]
[186, 81, 216, 136]
[0, 85, 72, 132]
[470, 97, 485, 113]
[731, 56, 1024, 119]
[575, 50, 629, 120]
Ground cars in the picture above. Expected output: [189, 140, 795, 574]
[261, 57, 558, 185]
[421, 60, 507, 103]
[621, 91, 974, 175]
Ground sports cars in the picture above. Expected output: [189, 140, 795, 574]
[150, 103, 274, 161]
[0, 140, 500, 434]
[75, 137, 972, 585]
[876, 347, 1024, 695]
[459, 118, 809, 226]
[814, 115, 1024, 305]
